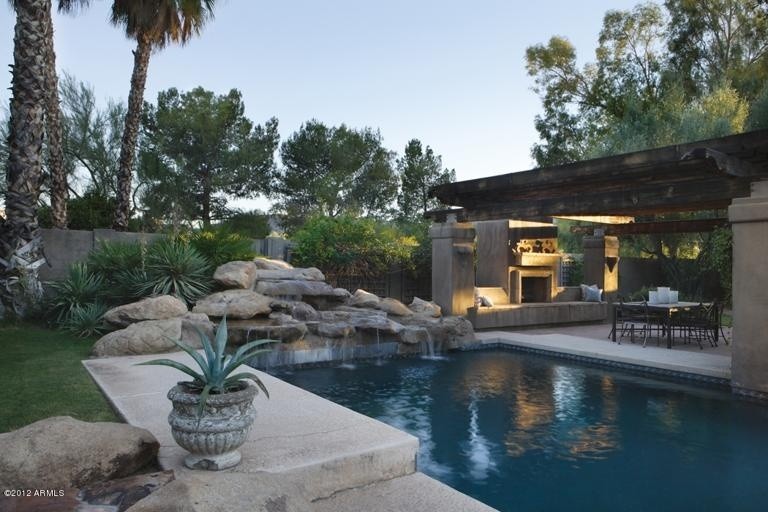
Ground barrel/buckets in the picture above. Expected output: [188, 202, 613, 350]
[656, 287, 671, 304]
[671, 291, 679, 304]
[649, 291, 657, 304]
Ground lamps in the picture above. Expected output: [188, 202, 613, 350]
[603, 254, 620, 273]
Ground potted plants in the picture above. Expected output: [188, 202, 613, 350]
[127, 306, 283, 472]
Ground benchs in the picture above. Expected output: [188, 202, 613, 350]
[465, 298, 609, 332]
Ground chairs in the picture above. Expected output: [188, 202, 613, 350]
[605, 295, 731, 349]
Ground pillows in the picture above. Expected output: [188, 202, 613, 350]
[583, 285, 604, 303]
[474, 287, 483, 307]
[578, 282, 599, 300]
[480, 293, 494, 307]
